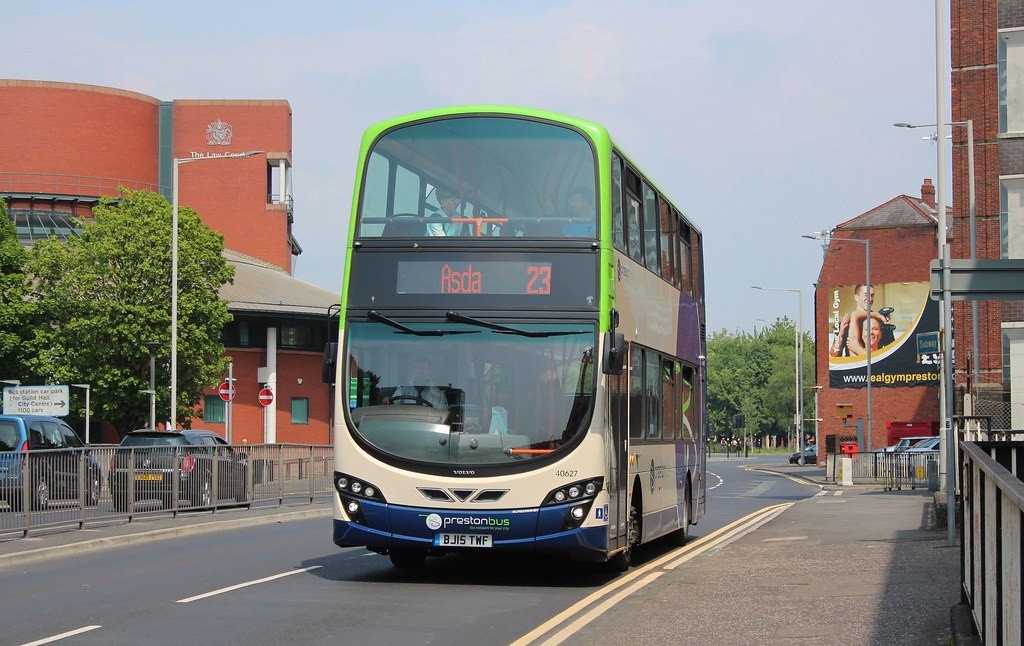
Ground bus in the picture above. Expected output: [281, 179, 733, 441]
[320, 104, 709, 576]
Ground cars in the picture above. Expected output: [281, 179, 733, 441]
[873, 436, 941, 477]
[789, 444, 817, 465]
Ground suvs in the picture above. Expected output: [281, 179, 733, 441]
[107, 428, 253, 511]
[0, 414, 104, 508]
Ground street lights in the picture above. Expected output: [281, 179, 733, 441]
[137, 391, 156, 430]
[167, 148, 267, 432]
[751, 286, 805, 466]
[799, 235, 873, 457]
[0, 378, 22, 387]
[894, 118, 981, 383]
[70, 383, 91, 447]
[755, 318, 799, 454]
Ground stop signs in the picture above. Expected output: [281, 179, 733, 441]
[219, 382, 235, 401]
[258, 388, 275, 406]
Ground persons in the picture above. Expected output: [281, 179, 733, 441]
[829, 284, 891, 356]
[382, 356, 448, 408]
[560, 187, 596, 238]
[718, 437, 744, 458]
[424, 189, 472, 237]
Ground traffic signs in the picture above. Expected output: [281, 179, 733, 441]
[3, 385, 69, 417]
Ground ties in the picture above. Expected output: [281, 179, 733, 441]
[415, 386, 424, 406]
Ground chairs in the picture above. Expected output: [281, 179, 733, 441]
[381, 213, 427, 237]
[547, 390, 664, 442]
